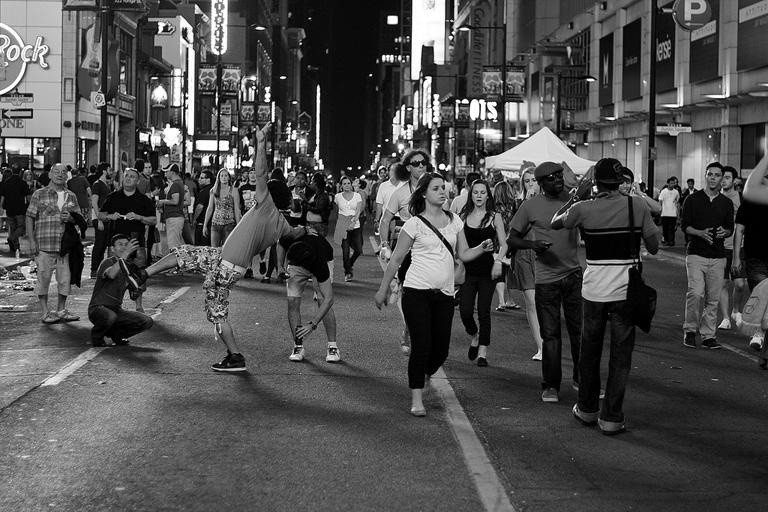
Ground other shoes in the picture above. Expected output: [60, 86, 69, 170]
[597, 416, 626, 431]
[749, 336, 763, 351]
[467, 341, 479, 361]
[683, 332, 697, 347]
[477, 357, 487, 367]
[423, 374, 429, 392]
[573, 403, 598, 423]
[731, 311, 742, 327]
[700, 337, 722, 348]
[410, 401, 426, 416]
[541, 388, 558, 401]
[111, 336, 129, 345]
[91, 326, 106, 347]
[572, 381, 605, 398]
[400, 330, 410, 355]
[718, 319, 732, 329]
[531, 351, 543, 360]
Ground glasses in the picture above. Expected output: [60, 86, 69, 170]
[546, 172, 564, 182]
[408, 158, 426, 168]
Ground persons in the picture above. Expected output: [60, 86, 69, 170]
[1, 122, 767, 435]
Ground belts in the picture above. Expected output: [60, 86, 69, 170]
[218, 256, 246, 276]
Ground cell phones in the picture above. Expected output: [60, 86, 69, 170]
[588, 176, 595, 185]
[279, 272, 290, 279]
[131, 232, 138, 246]
[544, 242, 553, 247]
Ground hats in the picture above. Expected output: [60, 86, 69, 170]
[534, 162, 564, 180]
[594, 158, 624, 185]
[162, 164, 180, 172]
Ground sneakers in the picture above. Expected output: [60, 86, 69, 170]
[40, 311, 62, 325]
[210, 349, 246, 371]
[119, 257, 146, 290]
[326, 345, 340, 361]
[289, 346, 305, 361]
[57, 310, 80, 321]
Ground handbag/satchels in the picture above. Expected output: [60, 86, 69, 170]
[627, 266, 657, 334]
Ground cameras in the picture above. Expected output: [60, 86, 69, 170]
[710, 225, 721, 237]
